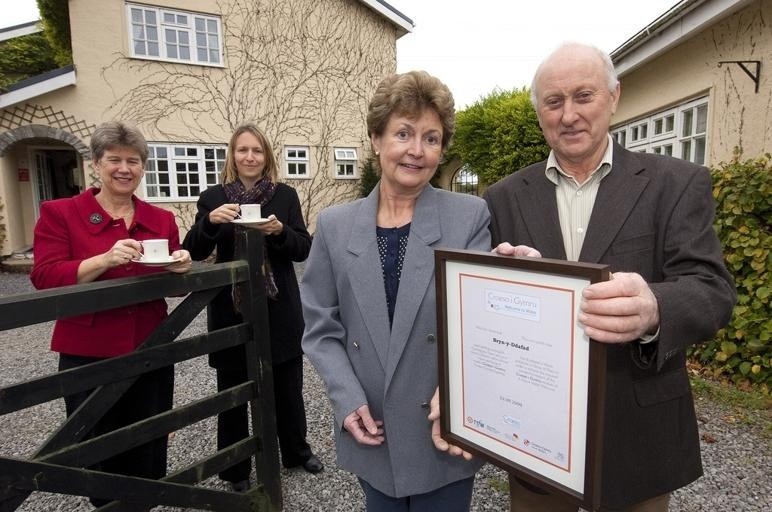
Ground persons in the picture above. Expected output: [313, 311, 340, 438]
[428, 45, 736, 512]
[30, 120, 193, 508]
[299, 71, 543, 512]
[182, 126, 322, 491]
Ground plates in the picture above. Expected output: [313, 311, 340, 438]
[131, 256, 181, 267]
[230, 218, 271, 226]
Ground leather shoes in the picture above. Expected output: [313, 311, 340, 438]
[231, 478, 249, 492]
[303, 453, 323, 473]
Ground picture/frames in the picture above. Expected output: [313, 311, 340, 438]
[433, 247, 610, 511]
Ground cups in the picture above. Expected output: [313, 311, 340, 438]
[236, 204, 261, 221]
[136, 239, 168, 261]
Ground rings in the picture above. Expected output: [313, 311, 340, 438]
[123, 254, 128, 259]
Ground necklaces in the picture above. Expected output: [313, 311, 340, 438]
[103, 208, 133, 227]
